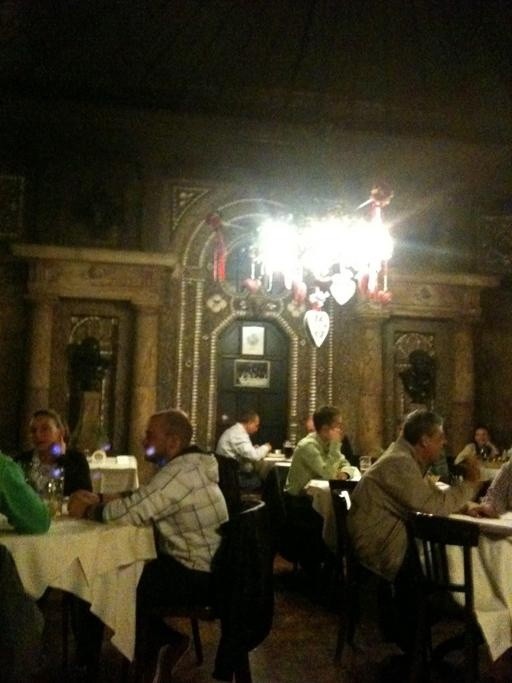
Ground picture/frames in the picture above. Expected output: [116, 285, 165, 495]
[235, 326, 271, 387]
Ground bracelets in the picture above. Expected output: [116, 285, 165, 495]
[97, 492, 105, 504]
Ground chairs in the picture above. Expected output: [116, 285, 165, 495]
[2, 455, 512, 683]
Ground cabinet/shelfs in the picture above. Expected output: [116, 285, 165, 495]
[218, 354, 284, 427]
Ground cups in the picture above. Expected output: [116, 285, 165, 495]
[360, 456, 371, 473]
[41, 477, 64, 520]
[283, 439, 294, 458]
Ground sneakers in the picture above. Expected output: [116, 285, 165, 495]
[138, 634, 192, 682]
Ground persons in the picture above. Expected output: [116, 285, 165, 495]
[1, 449, 52, 537]
[6, 407, 94, 518]
[210, 409, 273, 495]
[451, 422, 500, 481]
[344, 407, 499, 681]
[478, 455, 512, 521]
[280, 404, 357, 575]
[304, 412, 353, 462]
[66, 408, 232, 682]
